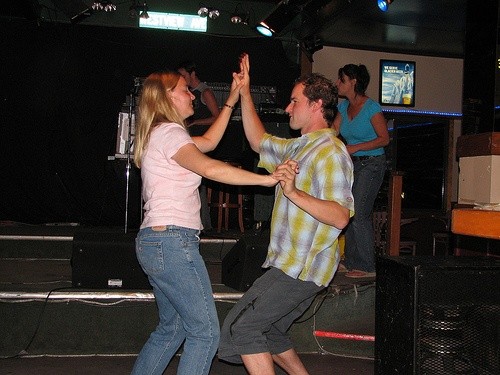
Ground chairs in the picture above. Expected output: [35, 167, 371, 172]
[373, 212, 417, 257]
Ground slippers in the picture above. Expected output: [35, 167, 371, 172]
[336, 263, 349, 272]
[345, 269, 376, 278]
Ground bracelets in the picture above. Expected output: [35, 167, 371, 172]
[223, 103, 234, 109]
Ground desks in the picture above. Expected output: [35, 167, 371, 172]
[451, 208, 500, 257]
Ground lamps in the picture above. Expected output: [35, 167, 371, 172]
[255, 0, 301, 39]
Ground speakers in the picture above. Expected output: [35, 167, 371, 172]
[71, 233, 154, 291]
[222, 237, 271, 291]
[375, 255, 500, 375]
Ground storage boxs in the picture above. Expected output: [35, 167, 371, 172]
[456, 132, 500, 156]
[457, 155, 500, 206]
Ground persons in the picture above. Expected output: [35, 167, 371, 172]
[330, 64, 390, 278]
[130, 62, 300, 375]
[218, 54, 355, 375]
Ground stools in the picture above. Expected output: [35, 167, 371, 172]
[205, 156, 250, 234]
[431, 233, 450, 256]
